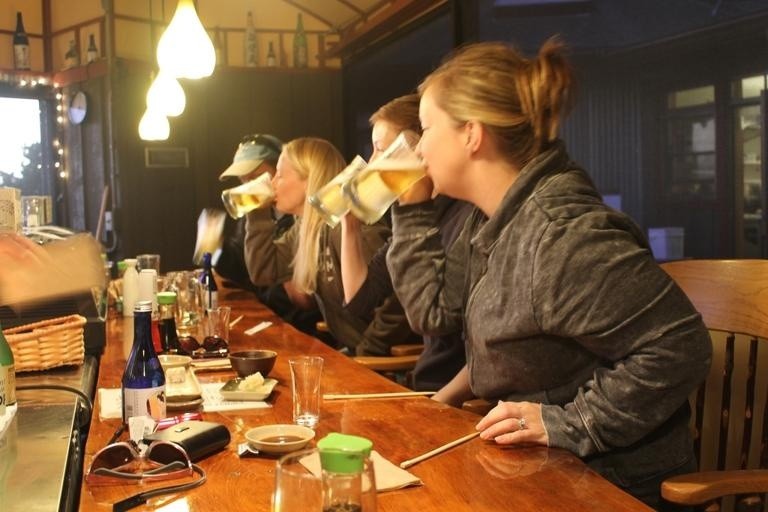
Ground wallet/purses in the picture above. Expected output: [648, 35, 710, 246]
[143, 419, 230, 461]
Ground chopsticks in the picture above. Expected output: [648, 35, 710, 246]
[399, 430, 488, 469]
[320, 390, 440, 399]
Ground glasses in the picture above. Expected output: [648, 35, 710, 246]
[238, 133, 280, 154]
[83, 441, 194, 487]
[167, 336, 230, 358]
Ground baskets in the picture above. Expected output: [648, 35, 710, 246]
[2, 313, 88, 371]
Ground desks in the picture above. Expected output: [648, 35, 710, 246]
[77, 262, 659, 511]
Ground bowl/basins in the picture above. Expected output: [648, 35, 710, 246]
[156, 354, 193, 371]
[245, 422, 316, 456]
[228, 349, 278, 378]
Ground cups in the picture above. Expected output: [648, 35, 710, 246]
[221, 172, 277, 221]
[122, 252, 222, 337]
[273, 446, 378, 511]
[206, 307, 230, 347]
[24, 198, 40, 227]
[345, 127, 429, 225]
[309, 154, 368, 228]
[288, 354, 326, 429]
[192, 208, 226, 265]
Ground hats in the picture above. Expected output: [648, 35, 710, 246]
[218, 133, 284, 183]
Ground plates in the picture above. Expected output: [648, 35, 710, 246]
[190, 357, 233, 372]
[221, 379, 278, 401]
[158, 366, 204, 412]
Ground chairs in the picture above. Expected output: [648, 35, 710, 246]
[352, 344, 425, 369]
[319, 322, 330, 332]
[462, 259, 766, 511]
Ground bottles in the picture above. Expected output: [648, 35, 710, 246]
[0, 328, 17, 405]
[268, 42, 275, 67]
[13, 10, 31, 72]
[316, 431, 373, 511]
[211, 25, 225, 66]
[157, 291, 186, 354]
[293, 11, 309, 69]
[244, 12, 258, 67]
[87, 34, 98, 62]
[0, 412, 20, 512]
[63, 40, 80, 67]
[121, 302, 167, 426]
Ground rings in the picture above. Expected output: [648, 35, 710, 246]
[520, 418, 525, 429]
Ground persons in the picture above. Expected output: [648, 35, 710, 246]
[343, 93, 476, 397]
[385, 31, 713, 512]
[219, 134, 338, 350]
[244, 137, 392, 357]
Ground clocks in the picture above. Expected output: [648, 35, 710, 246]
[67, 91, 89, 125]
